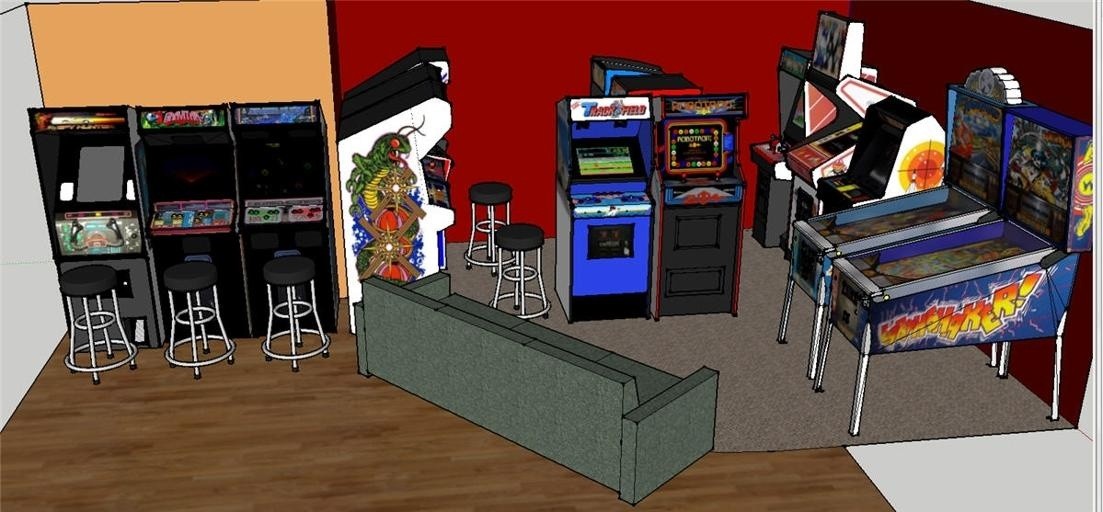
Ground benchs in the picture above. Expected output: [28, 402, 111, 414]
[354, 271, 721, 507]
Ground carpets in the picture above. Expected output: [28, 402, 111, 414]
[446, 228, 1076, 451]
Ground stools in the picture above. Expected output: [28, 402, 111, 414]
[464, 183, 552, 322]
[58, 255, 331, 384]
